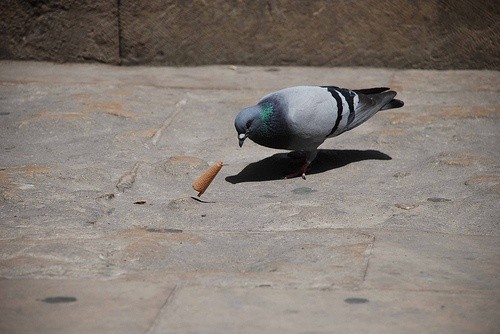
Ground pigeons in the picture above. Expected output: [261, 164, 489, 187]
[235, 85, 405, 179]
